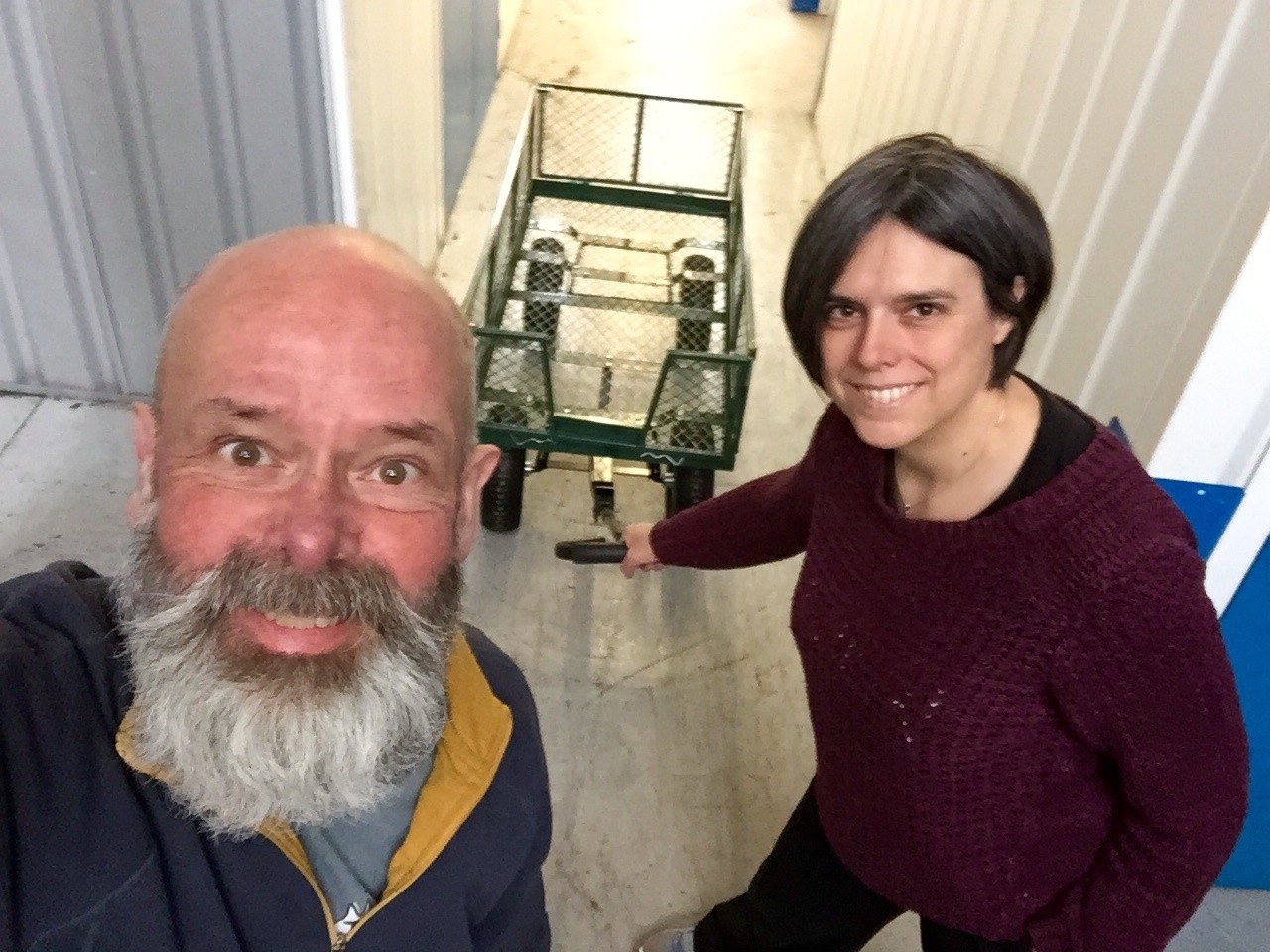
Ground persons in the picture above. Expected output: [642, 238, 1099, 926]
[616, 130, 1250, 952]
[0, 223, 554, 952]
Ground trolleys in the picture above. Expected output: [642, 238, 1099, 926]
[443, 82, 759, 566]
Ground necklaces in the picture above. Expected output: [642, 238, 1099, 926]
[905, 399, 1005, 511]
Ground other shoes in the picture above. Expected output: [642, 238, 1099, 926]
[638, 927, 694, 952]
